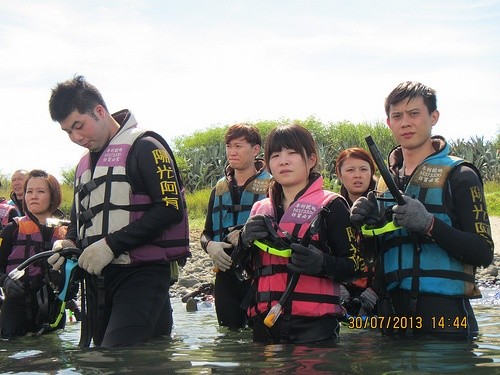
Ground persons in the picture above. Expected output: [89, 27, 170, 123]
[0, 169, 81, 338]
[349, 81, 494, 333]
[231, 125, 366, 345]
[48, 75, 191, 346]
[200, 122, 275, 331]
[336, 147, 383, 325]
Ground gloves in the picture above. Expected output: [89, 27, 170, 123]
[287, 242, 335, 280]
[47, 239, 76, 271]
[206, 240, 233, 272]
[350, 191, 381, 228]
[240, 214, 270, 244]
[391, 194, 433, 236]
[227, 226, 243, 247]
[77, 239, 113, 276]
